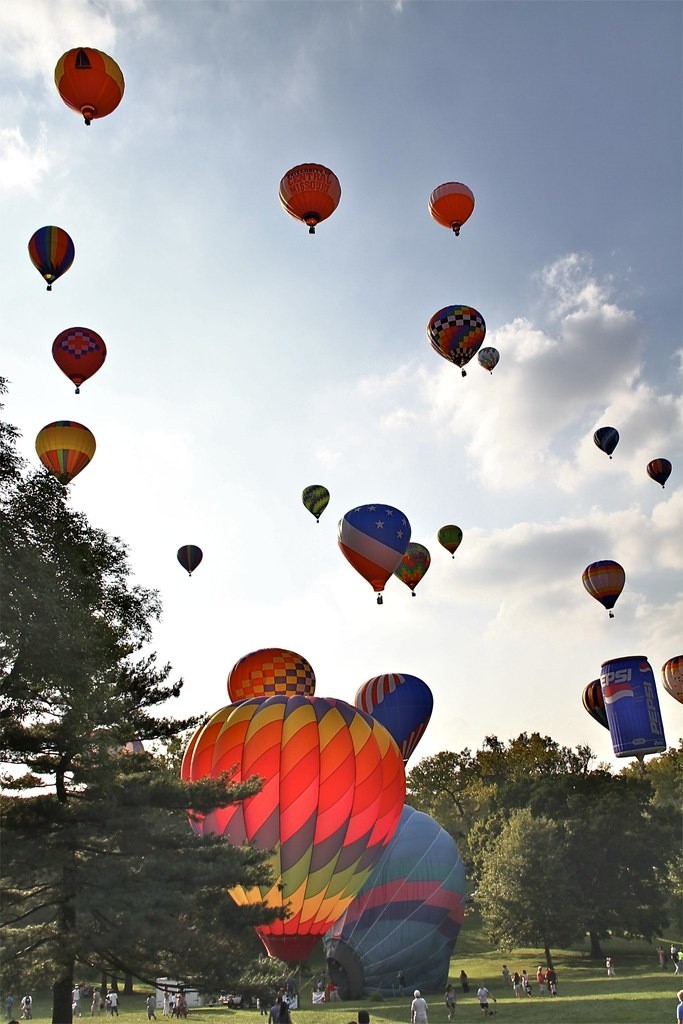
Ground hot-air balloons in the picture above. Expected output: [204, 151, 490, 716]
[54, 47, 125, 126]
[355, 673, 434, 770]
[35, 420, 97, 497]
[426, 305, 500, 377]
[52, 327, 107, 394]
[661, 654, 683, 704]
[338, 505, 412, 604]
[582, 678, 609, 730]
[302, 485, 330, 523]
[227, 648, 316, 704]
[428, 182, 475, 236]
[436, 525, 462, 559]
[180, 694, 467, 1002]
[593, 427, 619, 458]
[278, 163, 341, 234]
[394, 542, 430, 596]
[647, 458, 672, 488]
[177, 545, 203, 576]
[582, 560, 625, 618]
[28, 226, 75, 292]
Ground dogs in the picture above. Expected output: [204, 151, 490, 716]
[489, 1010, 498, 1015]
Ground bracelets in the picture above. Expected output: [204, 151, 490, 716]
[411, 1017, 413, 1018]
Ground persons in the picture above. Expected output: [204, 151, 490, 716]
[511, 972, 520, 999]
[670, 944, 683, 975]
[544, 967, 558, 997]
[606, 953, 615, 977]
[163, 986, 187, 1019]
[502, 965, 510, 989]
[71, 984, 119, 1018]
[145, 994, 156, 1021]
[460, 970, 468, 993]
[519, 970, 531, 998]
[656, 947, 666, 970]
[20, 993, 31, 1019]
[445, 985, 456, 1021]
[410, 990, 428, 1024]
[676, 990, 683, 1024]
[258, 980, 369, 1024]
[4, 992, 12, 1019]
[477, 983, 496, 1016]
[398, 971, 405, 996]
[536, 966, 545, 997]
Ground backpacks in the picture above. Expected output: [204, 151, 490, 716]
[25, 996, 30, 1005]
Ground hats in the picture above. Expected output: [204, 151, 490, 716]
[75, 984, 79, 988]
[414, 990, 420, 996]
